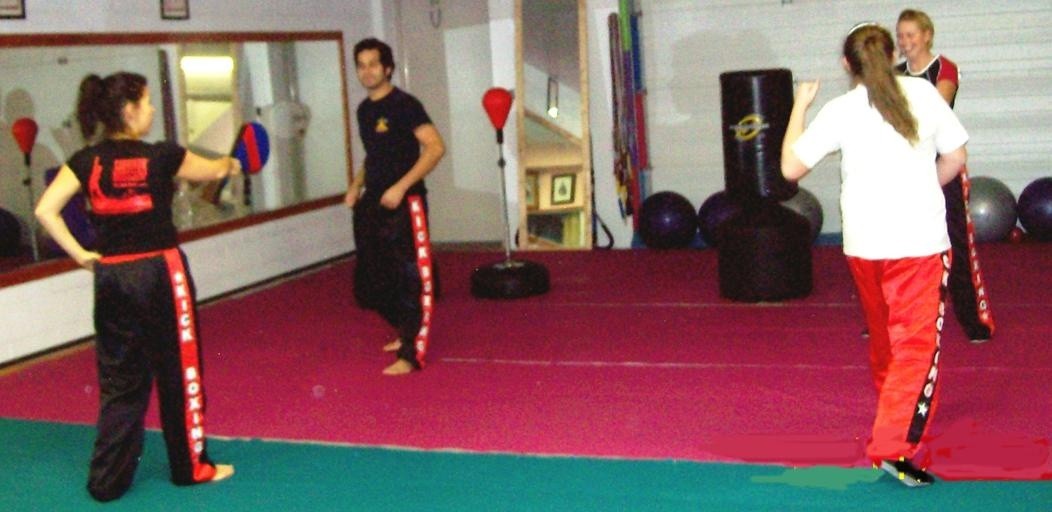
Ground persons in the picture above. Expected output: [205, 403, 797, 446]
[345, 38, 443, 376]
[35, 71, 243, 503]
[781, 21, 971, 487]
[892, 12, 996, 345]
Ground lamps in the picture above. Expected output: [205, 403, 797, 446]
[546, 74, 558, 119]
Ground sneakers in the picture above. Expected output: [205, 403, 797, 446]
[871, 452, 935, 488]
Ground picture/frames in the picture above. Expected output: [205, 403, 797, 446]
[160, 0, 190, 19]
[523, 168, 541, 210]
[551, 172, 576, 206]
[0, 1, 26, 19]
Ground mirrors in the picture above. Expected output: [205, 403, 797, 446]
[515, 0, 594, 252]
[0, 32, 353, 287]
